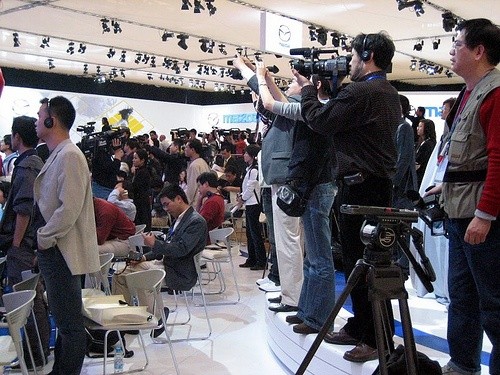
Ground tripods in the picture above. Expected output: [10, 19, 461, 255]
[295, 248, 417, 375]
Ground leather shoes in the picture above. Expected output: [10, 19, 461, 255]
[268, 302, 299, 312]
[324, 326, 360, 344]
[343, 340, 393, 362]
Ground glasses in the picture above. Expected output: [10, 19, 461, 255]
[451, 37, 475, 48]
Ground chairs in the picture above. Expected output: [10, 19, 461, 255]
[0, 198, 244, 375]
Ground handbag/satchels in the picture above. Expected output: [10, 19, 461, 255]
[275, 178, 307, 216]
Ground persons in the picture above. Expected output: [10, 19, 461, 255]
[424, 18, 500, 375]
[0, 32, 457, 375]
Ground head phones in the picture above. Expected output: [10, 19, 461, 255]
[44, 99, 53, 128]
[361, 35, 370, 62]
[206, 185, 223, 197]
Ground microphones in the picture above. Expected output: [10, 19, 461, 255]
[289, 47, 320, 58]
[411, 239, 436, 282]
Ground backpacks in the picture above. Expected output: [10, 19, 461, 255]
[372, 343, 443, 374]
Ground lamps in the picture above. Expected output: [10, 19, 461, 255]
[13, 0, 463, 96]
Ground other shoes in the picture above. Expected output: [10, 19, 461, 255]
[286, 315, 302, 323]
[11, 347, 51, 369]
[268, 294, 282, 302]
[239, 262, 268, 270]
[293, 322, 320, 333]
[440, 361, 479, 374]
[256, 277, 282, 291]
[150, 306, 169, 337]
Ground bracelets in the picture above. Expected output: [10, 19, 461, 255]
[258, 81, 267, 86]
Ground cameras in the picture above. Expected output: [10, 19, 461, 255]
[411, 186, 447, 236]
[127, 251, 143, 262]
[227, 52, 242, 65]
